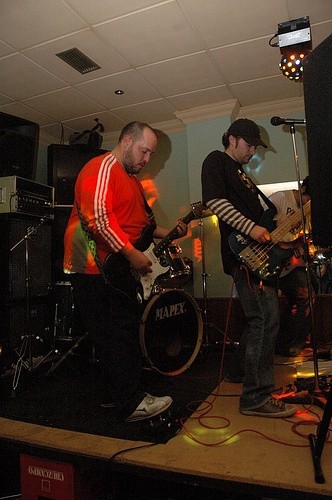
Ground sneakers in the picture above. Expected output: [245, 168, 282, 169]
[242, 397, 297, 418]
[127, 395, 173, 423]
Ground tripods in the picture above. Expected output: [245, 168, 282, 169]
[202, 218, 240, 348]
[0, 219, 64, 399]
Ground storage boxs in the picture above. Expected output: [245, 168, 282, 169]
[19, 446, 107, 500]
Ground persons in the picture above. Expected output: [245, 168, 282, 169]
[268, 176, 318, 357]
[63, 122, 188, 422]
[201, 118, 298, 418]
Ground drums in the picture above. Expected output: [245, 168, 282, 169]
[48, 279, 80, 344]
[156, 236, 191, 285]
[138, 284, 204, 377]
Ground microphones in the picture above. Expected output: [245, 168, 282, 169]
[271, 116, 306, 126]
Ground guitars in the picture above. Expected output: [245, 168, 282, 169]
[102, 202, 210, 304]
[228, 199, 312, 285]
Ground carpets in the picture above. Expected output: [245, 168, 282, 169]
[0, 345, 242, 445]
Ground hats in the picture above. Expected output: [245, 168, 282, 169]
[228, 119, 269, 149]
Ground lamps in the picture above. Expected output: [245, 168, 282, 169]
[268, 16, 312, 83]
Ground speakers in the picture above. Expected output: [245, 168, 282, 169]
[0, 213, 52, 301]
[1, 300, 52, 371]
